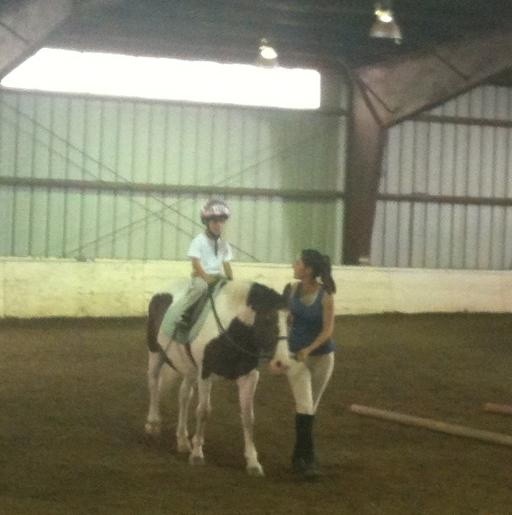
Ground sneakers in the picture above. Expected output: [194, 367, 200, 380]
[177, 314, 187, 328]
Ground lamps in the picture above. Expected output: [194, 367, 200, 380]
[368, 0, 402, 41]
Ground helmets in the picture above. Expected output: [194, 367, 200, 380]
[202, 200, 231, 220]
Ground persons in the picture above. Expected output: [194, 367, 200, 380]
[171, 199, 235, 330]
[279, 248, 338, 481]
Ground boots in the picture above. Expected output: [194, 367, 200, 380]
[292, 413, 319, 479]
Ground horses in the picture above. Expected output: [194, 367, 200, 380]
[143, 277, 296, 480]
